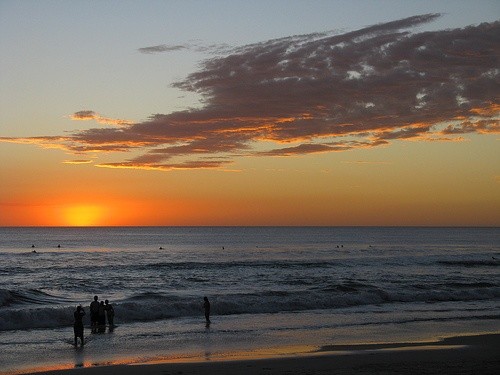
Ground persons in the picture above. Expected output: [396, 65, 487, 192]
[104, 300, 115, 332]
[89, 295, 99, 334]
[203, 296, 210, 328]
[73, 305, 85, 349]
[98, 301, 106, 334]
